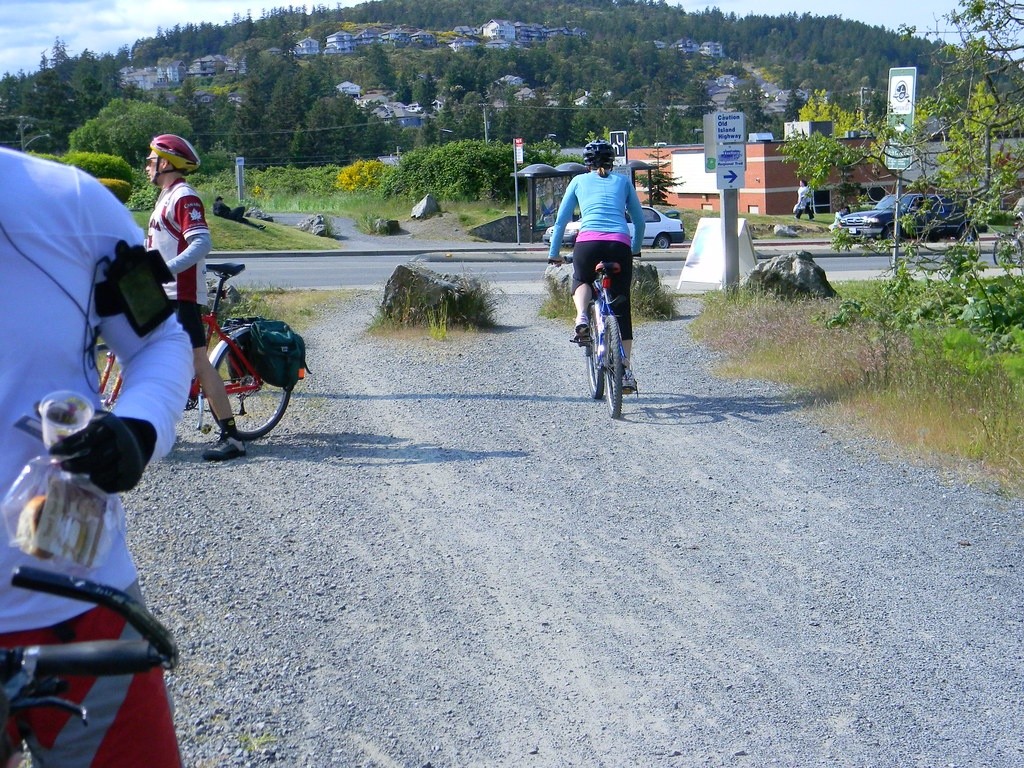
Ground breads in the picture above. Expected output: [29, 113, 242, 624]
[16, 496, 69, 559]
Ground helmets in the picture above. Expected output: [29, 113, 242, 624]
[150, 134, 200, 172]
[583, 139, 616, 166]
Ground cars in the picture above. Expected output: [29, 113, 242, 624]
[838, 192, 988, 243]
[542, 206, 686, 248]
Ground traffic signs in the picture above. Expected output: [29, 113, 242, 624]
[716, 143, 745, 191]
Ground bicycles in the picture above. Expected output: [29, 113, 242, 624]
[1, 566, 180, 768]
[547, 253, 643, 419]
[95, 261, 314, 442]
[992, 211, 1024, 268]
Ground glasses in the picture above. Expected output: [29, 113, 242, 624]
[146, 153, 162, 166]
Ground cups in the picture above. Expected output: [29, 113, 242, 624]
[38, 389, 96, 451]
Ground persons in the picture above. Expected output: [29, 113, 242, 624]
[548, 140, 645, 393]
[145, 135, 246, 459]
[213, 196, 266, 230]
[0, 145, 194, 768]
[794, 179, 814, 221]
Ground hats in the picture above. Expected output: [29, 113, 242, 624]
[216, 196, 224, 201]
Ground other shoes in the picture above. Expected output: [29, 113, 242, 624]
[575, 324, 590, 336]
[808, 218, 815, 221]
[622, 375, 637, 386]
[259, 225, 266, 229]
[795, 217, 799, 221]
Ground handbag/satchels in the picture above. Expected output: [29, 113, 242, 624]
[225, 317, 268, 384]
[250, 319, 313, 391]
[793, 197, 804, 213]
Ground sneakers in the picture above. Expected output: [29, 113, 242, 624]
[201, 432, 247, 460]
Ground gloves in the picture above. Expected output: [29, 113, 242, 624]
[548, 255, 563, 267]
[48, 403, 158, 494]
